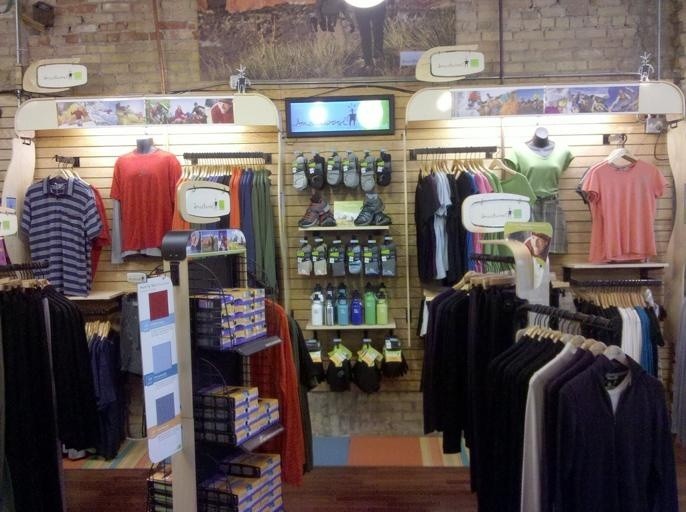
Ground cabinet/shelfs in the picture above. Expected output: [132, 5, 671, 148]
[146, 229, 284, 512]
[298, 226, 396, 330]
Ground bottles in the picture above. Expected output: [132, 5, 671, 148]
[308, 282, 389, 326]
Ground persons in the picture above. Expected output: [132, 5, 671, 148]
[108, 139, 189, 263]
[524, 230, 552, 260]
[505, 123, 576, 256]
[56, 98, 234, 126]
[354, 3, 387, 72]
[467, 87, 638, 115]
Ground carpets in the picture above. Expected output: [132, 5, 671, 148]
[312, 437, 471, 467]
[63, 437, 168, 469]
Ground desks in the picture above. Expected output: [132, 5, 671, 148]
[67, 292, 125, 321]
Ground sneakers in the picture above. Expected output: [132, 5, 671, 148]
[299, 192, 336, 228]
[354, 193, 391, 226]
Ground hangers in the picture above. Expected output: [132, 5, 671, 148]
[604, 133, 639, 163]
[49, 155, 90, 186]
[85, 309, 112, 338]
[573, 279, 658, 309]
[420, 145, 516, 179]
[523, 303, 629, 366]
[452, 254, 556, 291]
[0, 261, 51, 291]
[180, 152, 266, 179]
